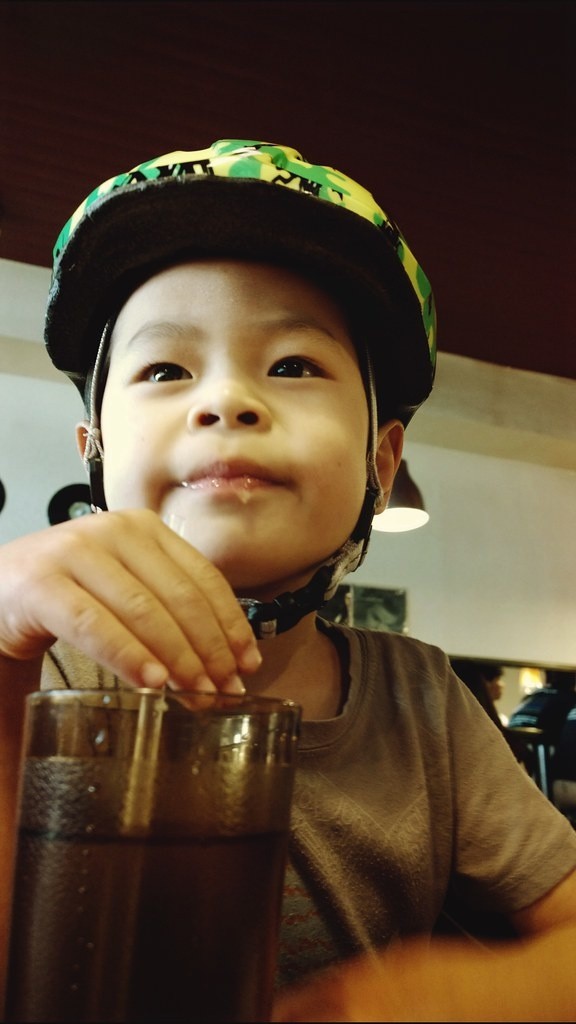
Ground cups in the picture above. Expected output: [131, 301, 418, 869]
[0, 688, 303, 1024]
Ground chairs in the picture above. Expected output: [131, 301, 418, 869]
[501, 727, 576, 833]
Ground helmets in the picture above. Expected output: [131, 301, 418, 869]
[43, 140, 439, 428]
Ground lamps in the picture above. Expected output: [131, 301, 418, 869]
[370, 458, 430, 533]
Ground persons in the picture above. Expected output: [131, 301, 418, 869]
[363, 599, 400, 630]
[0, 138, 576, 1024]
